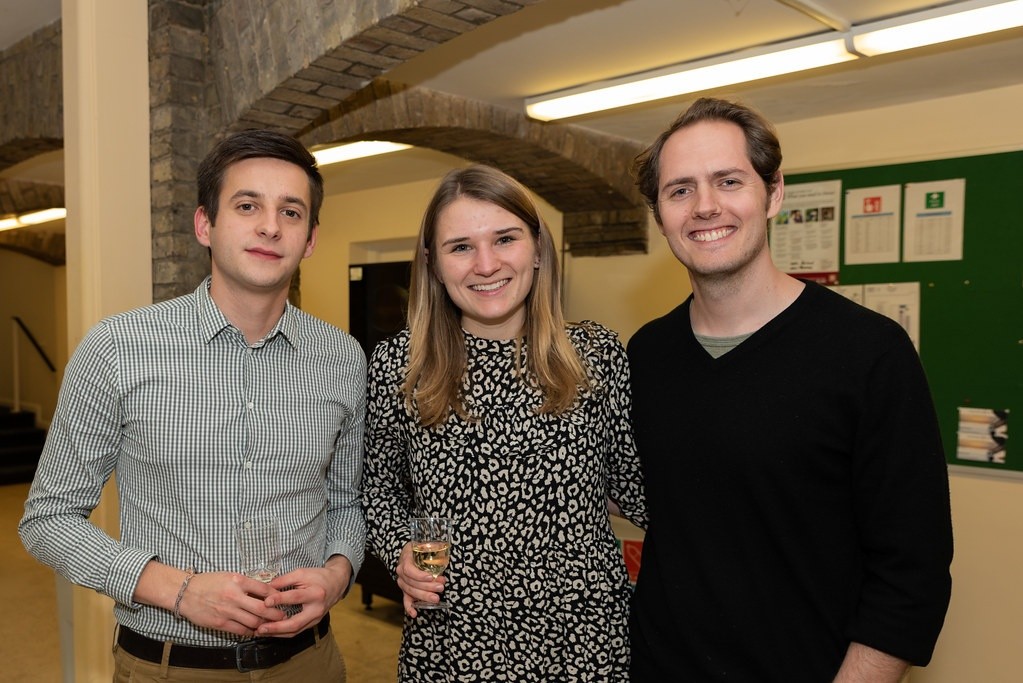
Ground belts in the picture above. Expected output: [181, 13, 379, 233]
[116, 612, 333, 670]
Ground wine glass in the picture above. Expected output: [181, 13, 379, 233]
[409, 518, 451, 609]
[233, 515, 281, 603]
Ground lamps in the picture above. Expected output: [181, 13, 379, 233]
[310, 135, 417, 172]
[523, 0, 1023, 126]
[0, 203, 68, 233]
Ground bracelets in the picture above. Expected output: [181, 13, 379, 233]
[173, 572, 196, 619]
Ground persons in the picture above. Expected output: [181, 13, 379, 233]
[359, 165, 654, 683]
[619, 97, 957, 683]
[12, 126, 369, 683]
